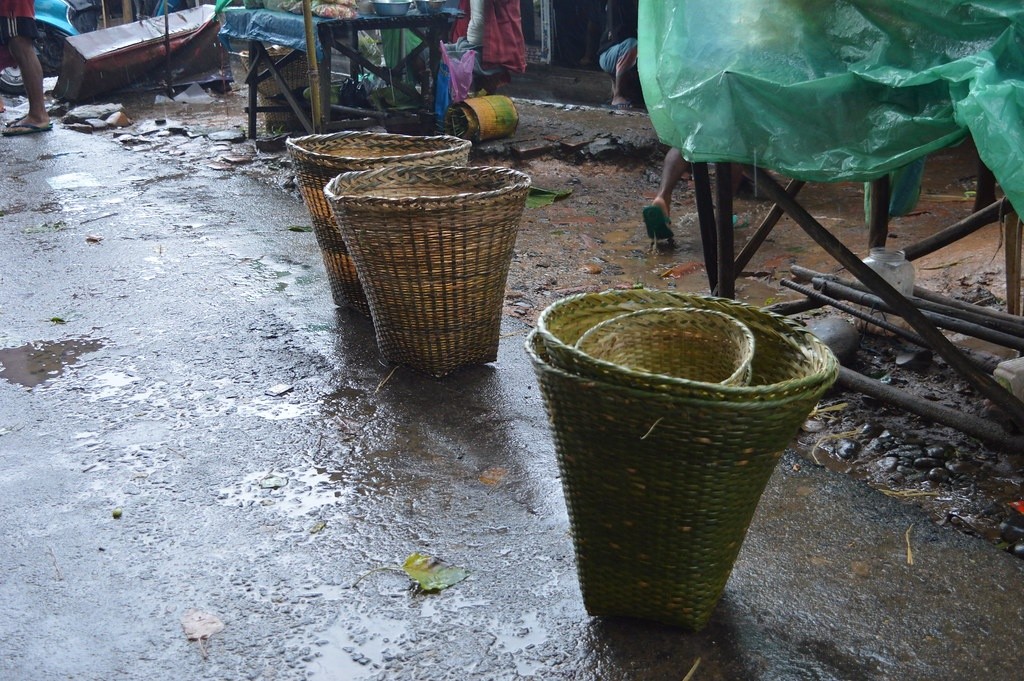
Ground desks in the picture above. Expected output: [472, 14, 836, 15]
[219, 6, 465, 136]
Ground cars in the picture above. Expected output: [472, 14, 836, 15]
[0, 0, 100, 95]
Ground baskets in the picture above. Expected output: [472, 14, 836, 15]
[286, 130, 473, 320]
[525, 288, 840, 629]
[323, 165, 531, 377]
[239, 46, 313, 134]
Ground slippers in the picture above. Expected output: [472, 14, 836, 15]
[643, 205, 673, 241]
[608, 101, 633, 110]
[6, 118, 53, 126]
[1, 123, 51, 136]
[732, 215, 748, 228]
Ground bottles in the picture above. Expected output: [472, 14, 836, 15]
[856, 247, 914, 338]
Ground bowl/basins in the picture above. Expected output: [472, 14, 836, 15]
[414, 0, 446, 14]
[369, 0, 414, 15]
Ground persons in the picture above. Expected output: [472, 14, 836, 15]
[597, 0, 638, 110]
[0, 0, 56, 136]
[642, 147, 691, 240]
